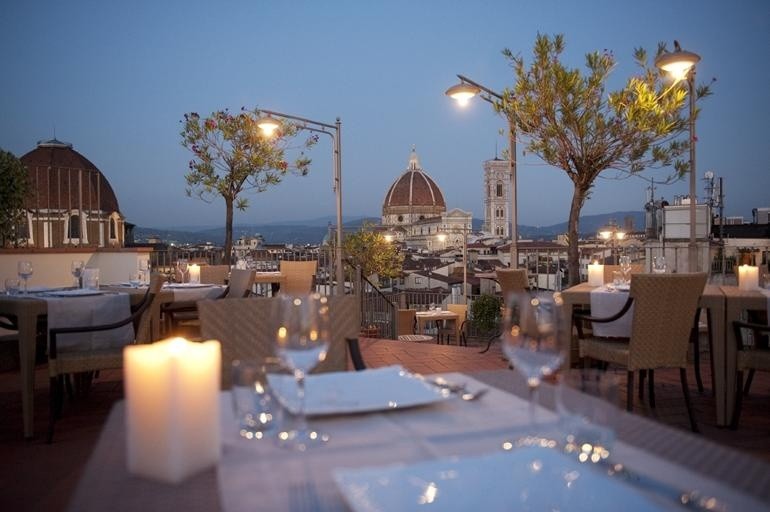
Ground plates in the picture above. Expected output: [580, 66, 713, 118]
[163, 283, 215, 288]
[330, 441, 683, 512]
[266, 363, 459, 418]
[18, 286, 64, 292]
[43, 288, 108, 297]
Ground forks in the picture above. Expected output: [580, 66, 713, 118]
[421, 376, 491, 404]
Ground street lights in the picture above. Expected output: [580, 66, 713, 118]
[654, 40, 702, 271]
[256, 107, 345, 297]
[438, 223, 469, 304]
[445, 73, 520, 269]
[599, 224, 627, 265]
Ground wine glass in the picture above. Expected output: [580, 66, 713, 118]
[135, 254, 150, 287]
[70, 259, 83, 287]
[176, 258, 189, 283]
[263, 293, 331, 453]
[618, 256, 632, 283]
[500, 288, 568, 424]
[650, 254, 667, 274]
[16, 260, 33, 296]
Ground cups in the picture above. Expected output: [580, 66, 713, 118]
[555, 367, 620, 447]
[128, 271, 141, 286]
[228, 355, 308, 441]
[3, 279, 20, 297]
[612, 270, 623, 285]
[82, 266, 99, 289]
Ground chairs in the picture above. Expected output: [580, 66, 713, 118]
[496, 267, 557, 376]
[174, 265, 230, 285]
[161, 270, 257, 338]
[46, 271, 167, 438]
[588, 264, 645, 287]
[732, 319, 770, 430]
[572, 271, 708, 433]
[197, 294, 367, 389]
[277, 259, 318, 295]
[439, 304, 468, 347]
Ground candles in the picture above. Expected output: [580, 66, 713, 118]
[587, 261, 604, 287]
[738, 266, 759, 291]
[123, 335, 222, 485]
